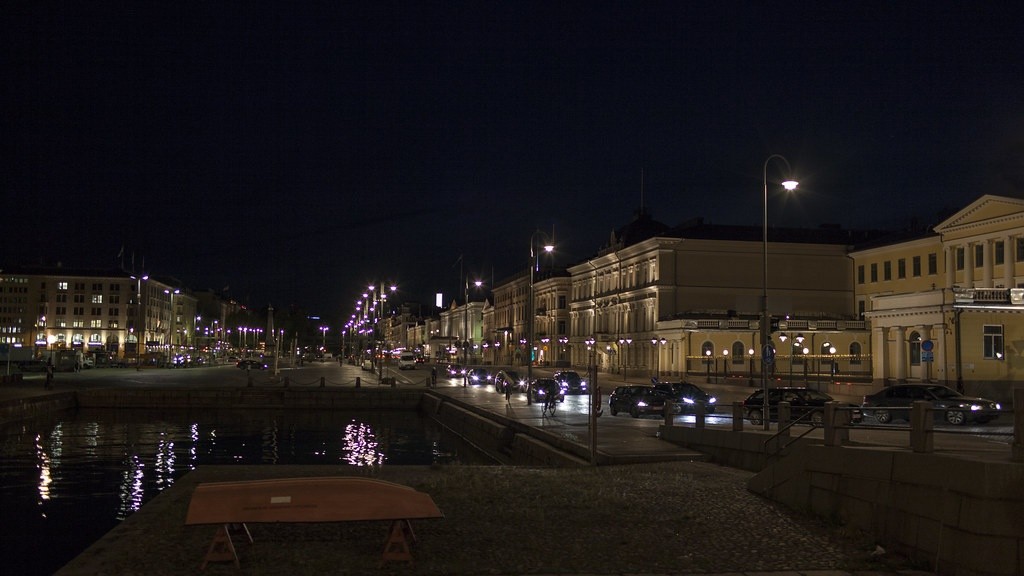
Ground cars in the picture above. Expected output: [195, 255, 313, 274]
[742, 385, 863, 428]
[861, 379, 1002, 425]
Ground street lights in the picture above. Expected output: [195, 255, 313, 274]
[37, 276, 284, 368]
[319, 152, 836, 429]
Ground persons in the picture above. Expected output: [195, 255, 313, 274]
[431, 363, 824, 410]
